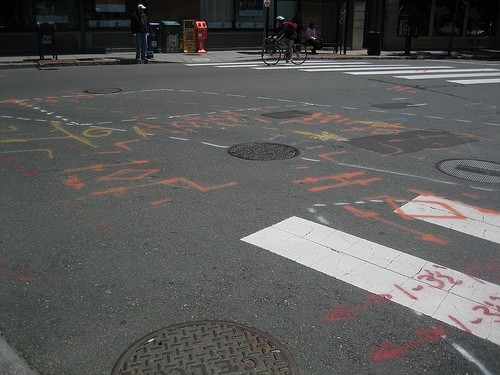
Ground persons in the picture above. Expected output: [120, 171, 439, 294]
[305, 22, 321, 54]
[268, 16, 297, 62]
[131, 3, 150, 61]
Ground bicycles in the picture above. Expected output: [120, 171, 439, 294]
[261, 38, 308, 66]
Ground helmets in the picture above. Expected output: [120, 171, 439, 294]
[276, 16, 285, 21]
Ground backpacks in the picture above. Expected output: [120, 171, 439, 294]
[283, 21, 297, 31]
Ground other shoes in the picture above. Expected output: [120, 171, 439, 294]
[311, 49, 318, 54]
[143, 57, 147, 61]
[286, 58, 292, 63]
[136, 57, 140, 61]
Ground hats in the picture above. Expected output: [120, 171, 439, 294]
[137, 4, 146, 9]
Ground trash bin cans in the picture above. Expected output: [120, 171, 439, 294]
[37, 21, 58, 60]
[367, 32, 383, 55]
[195, 21, 208, 53]
[180, 19, 196, 53]
[161, 20, 181, 53]
[148, 22, 161, 53]
